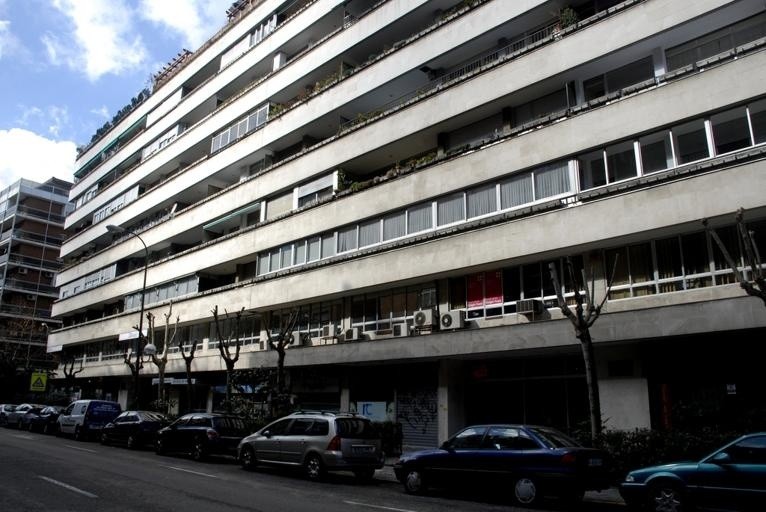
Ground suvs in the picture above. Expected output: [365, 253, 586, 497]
[236, 409, 387, 482]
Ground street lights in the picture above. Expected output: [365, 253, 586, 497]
[106, 225, 156, 412]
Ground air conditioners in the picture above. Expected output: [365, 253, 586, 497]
[287, 331, 302, 347]
[515, 299, 544, 315]
[320, 324, 361, 342]
[392, 308, 465, 337]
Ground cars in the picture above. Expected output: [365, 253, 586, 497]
[619, 431, 766, 512]
[394, 422, 611, 507]
[102, 409, 246, 463]
[0, 400, 121, 437]
